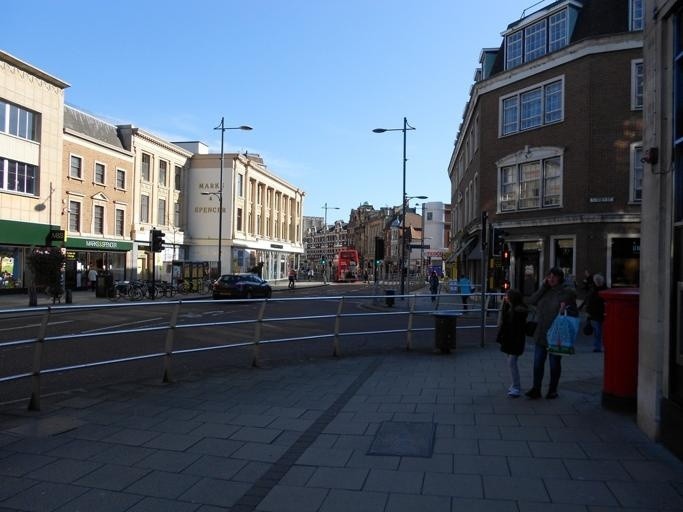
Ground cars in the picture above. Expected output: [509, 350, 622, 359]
[212, 272, 272, 300]
[576, 283, 634, 322]
[105, 278, 215, 303]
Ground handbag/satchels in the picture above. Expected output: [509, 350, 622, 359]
[543, 307, 582, 358]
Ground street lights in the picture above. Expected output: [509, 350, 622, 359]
[320, 203, 340, 282]
[200, 117, 252, 275]
[372, 116, 427, 301]
[414, 201, 432, 276]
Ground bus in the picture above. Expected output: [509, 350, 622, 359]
[331, 249, 358, 283]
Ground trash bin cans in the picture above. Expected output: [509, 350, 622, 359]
[429, 312, 463, 355]
[597, 287, 640, 413]
[384, 289, 394, 307]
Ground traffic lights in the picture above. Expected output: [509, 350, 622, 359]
[152, 230, 164, 252]
[321, 256, 325, 264]
[501, 249, 510, 269]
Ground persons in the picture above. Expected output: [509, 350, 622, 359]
[522, 267, 579, 400]
[503, 279, 509, 289]
[494, 289, 528, 398]
[576, 272, 608, 353]
[87, 267, 97, 292]
[287, 269, 294, 288]
[428, 271, 438, 307]
[363, 269, 370, 284]
[456, 273, 472, 313]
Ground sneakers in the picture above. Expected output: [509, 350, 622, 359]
[506, 384, 559, 401]
